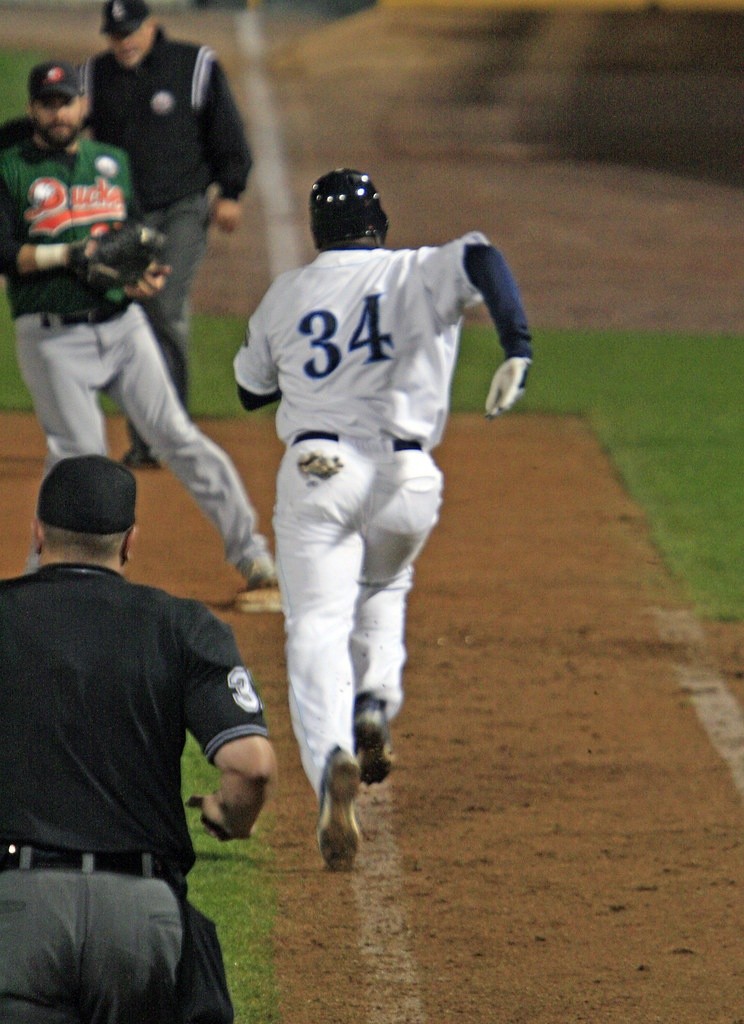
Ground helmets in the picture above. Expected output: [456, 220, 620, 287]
[309, 168, 389, 248]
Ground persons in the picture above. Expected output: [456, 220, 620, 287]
[233, 167, 531, 869]
[75, 0, 254, 472]
[0, 453, 277, 1024]
[0, 59, 284, 612]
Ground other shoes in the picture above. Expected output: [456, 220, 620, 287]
[122, 445, 162, 471]
[316, 745, 363, 872]
[353, 693, 394, 786]
[233, 559, 281, 614]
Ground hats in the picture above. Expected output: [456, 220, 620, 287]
[102, 0, 150, 38]
[36, 455, 136, 534]
[28, 62, 78, 101]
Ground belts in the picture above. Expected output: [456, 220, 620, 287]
[0, 840, 162, 879]
[293, 430, 422, 451]
[43, 297, 133, 327]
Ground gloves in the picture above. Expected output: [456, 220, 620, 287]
[485, 354, 530, 417]
